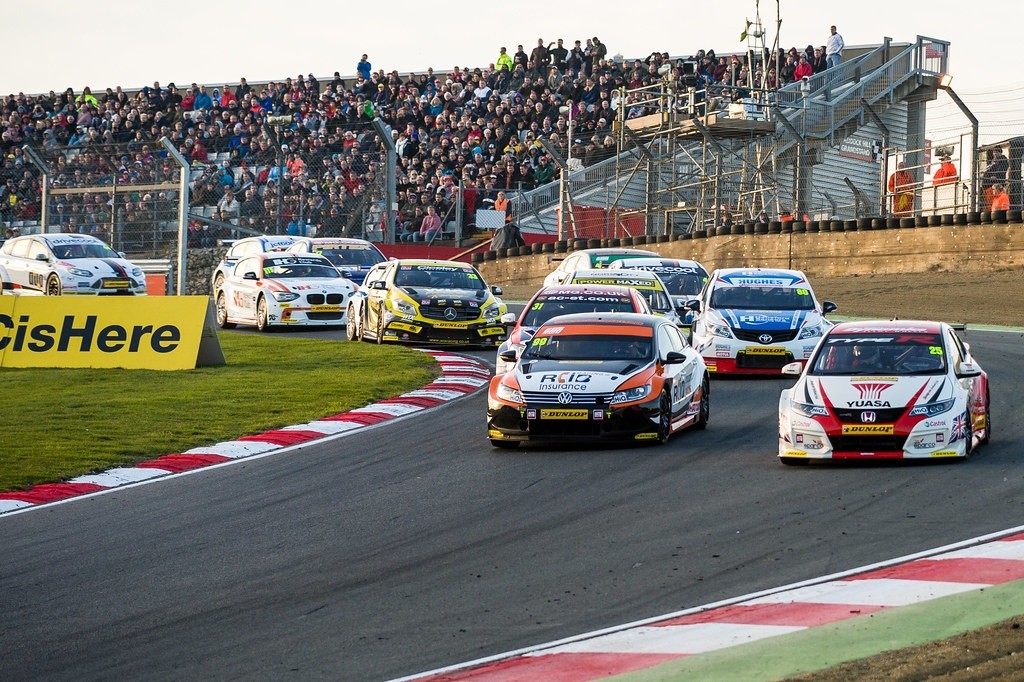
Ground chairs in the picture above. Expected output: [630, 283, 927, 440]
[2, 150, 457, 248]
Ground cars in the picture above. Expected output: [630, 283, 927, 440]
[683, 267, 837, 375]
[484, 314, 712, 449]
[212, 234, 307, 301]
[213, 254, 363, 332]
[492, 283, 656, 376]
[284, 236, 399, 286]
[610, 254, 715, 329]
[558, 268, 685, 329]
[774, 320, 992, 464]
[542, 248, 661, 294]
[345, 261, 392, 340]
[360, 261, 511, 347]
[0, 232, 147, 297]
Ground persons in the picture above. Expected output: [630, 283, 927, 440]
[611, 341, 645, 359]
[296, 267, 307, 276]
[887, 161, 916, 218]
[990, 185, 1010, 212]
[982, 145, 1008, 210]
[851, 345, 864, 370]
[672, 276, 692, 296]
[0, 25, 844, 252]
[933, 157, 958, 187]
